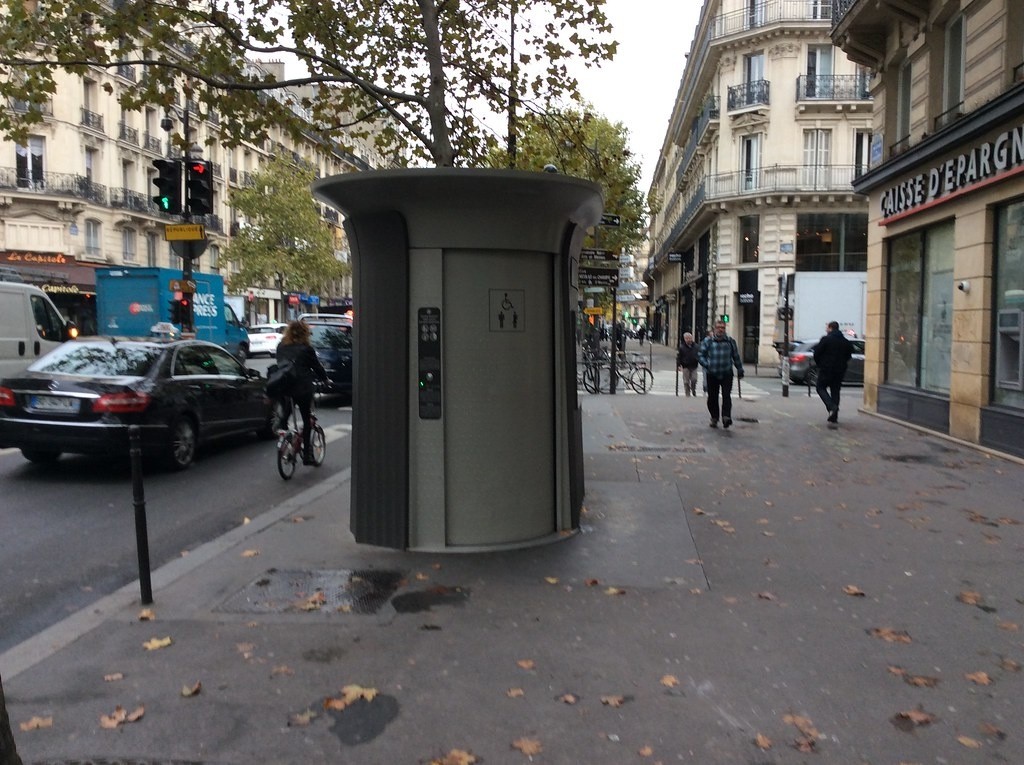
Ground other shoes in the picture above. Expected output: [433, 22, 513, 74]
[274, 422, 287, 430]
[303, 458, 316, 465]
[710, 418, 718, 427]
[829, 410, 838, 422]
[722, 416, 732, 428]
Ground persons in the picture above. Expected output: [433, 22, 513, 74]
[647, 327, 656, 344]
[814, 322, 850, 423]
[272, 321, 326, 466]
[697, 321, 744, 428]
[676, 332, 700, 396]
[699, 327, 715, 391]
[638, 325, 646, 345]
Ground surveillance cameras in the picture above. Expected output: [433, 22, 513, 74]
[958, 280, 970, 293]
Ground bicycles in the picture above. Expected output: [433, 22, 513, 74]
[277, 373, 332, 480]
[577, 341, 654, 396]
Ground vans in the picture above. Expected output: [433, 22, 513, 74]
[0, 281, 79, 374]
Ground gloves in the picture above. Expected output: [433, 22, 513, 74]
[737, 371, 744, 378]
[323, 376, 333, 388]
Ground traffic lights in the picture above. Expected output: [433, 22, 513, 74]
[167, 299, 190, 324]
[152, 159, 182, 215]
[189, 159, 215, 216]
[721, 315, 729, 323]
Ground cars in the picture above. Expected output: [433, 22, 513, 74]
[302, 321, 353, 398]
[245, 323, 291, 358]
[298, 313, 353, 325]
[0, 335, 285, 471]
[779, 338, 865, 386]
